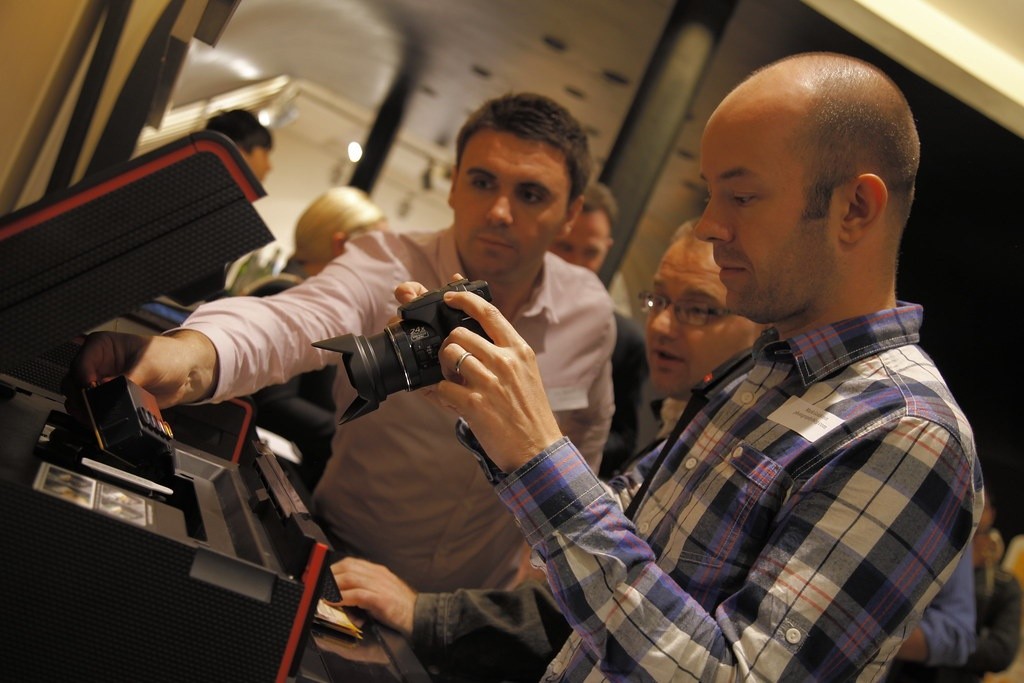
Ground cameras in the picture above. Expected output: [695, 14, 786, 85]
[311, 278, 493, 426]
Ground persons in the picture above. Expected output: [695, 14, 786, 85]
[549, 185, 648, 481]
[177, 106, 273, 306]
[329, 216, 774, 683]
[242, 186, 392, 492]
[62, 91, 618, 592]
[395, 53, 985, 683]
[888, 494, 1024, 683]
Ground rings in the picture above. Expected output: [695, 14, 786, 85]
[456, 352, 471, 375]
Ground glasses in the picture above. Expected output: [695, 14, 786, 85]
[638, 291, 730, 327]
